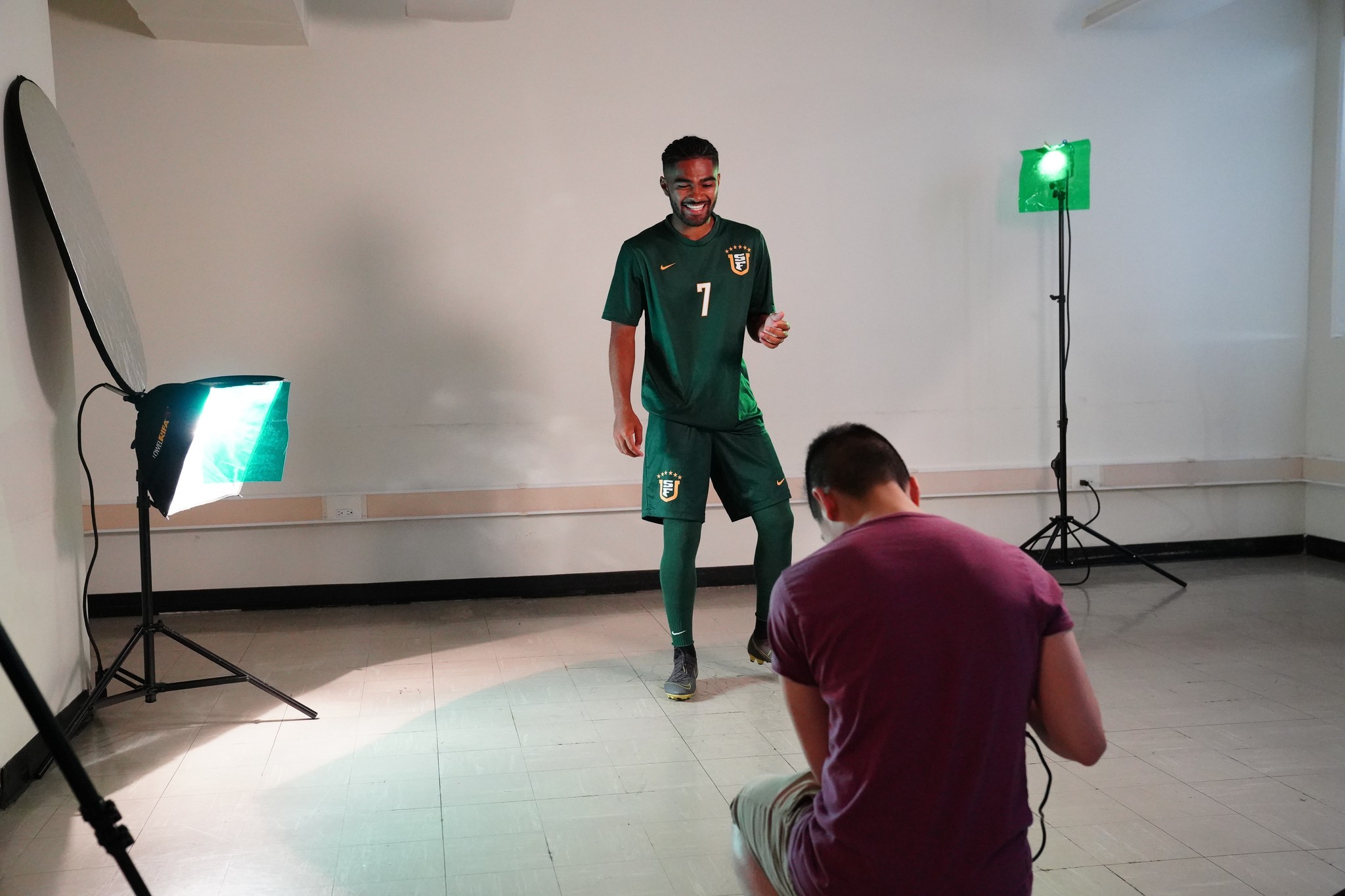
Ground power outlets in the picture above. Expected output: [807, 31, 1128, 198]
[1071, 465, 1100, 488]
[321, 496, 368, 521]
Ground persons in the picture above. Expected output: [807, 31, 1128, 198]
[600, 135, 795, 701]
[729, 424, 1106, 896]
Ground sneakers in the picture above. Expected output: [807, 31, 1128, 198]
[663, 647, 700, 701]
[745, 633, 772, 666]
